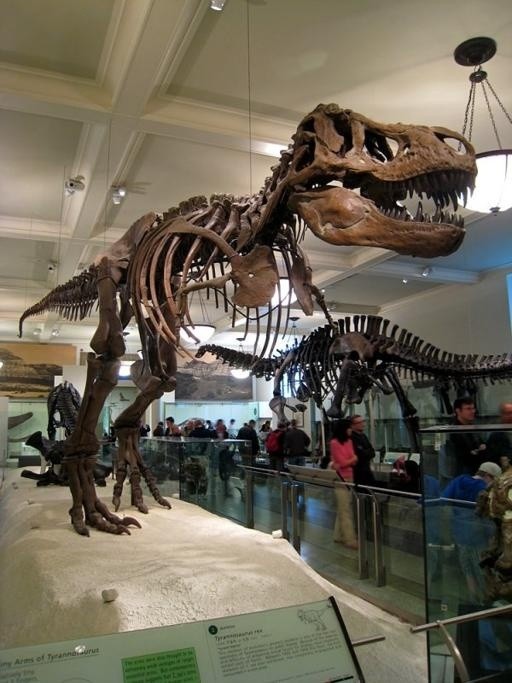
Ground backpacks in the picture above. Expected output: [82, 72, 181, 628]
[265, 431, 283, 452]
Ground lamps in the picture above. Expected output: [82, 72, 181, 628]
[179, 270, 217, 343]
[452, 36, 512, 215]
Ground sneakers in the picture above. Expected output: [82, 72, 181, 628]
[334, 539, 359, 550]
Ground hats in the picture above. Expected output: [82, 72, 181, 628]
[479, 461, 502, 477]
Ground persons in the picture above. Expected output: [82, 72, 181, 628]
[138, 417, 312, 499]
[103, 420, 118, 456]
[314, 397, 511, 612]
